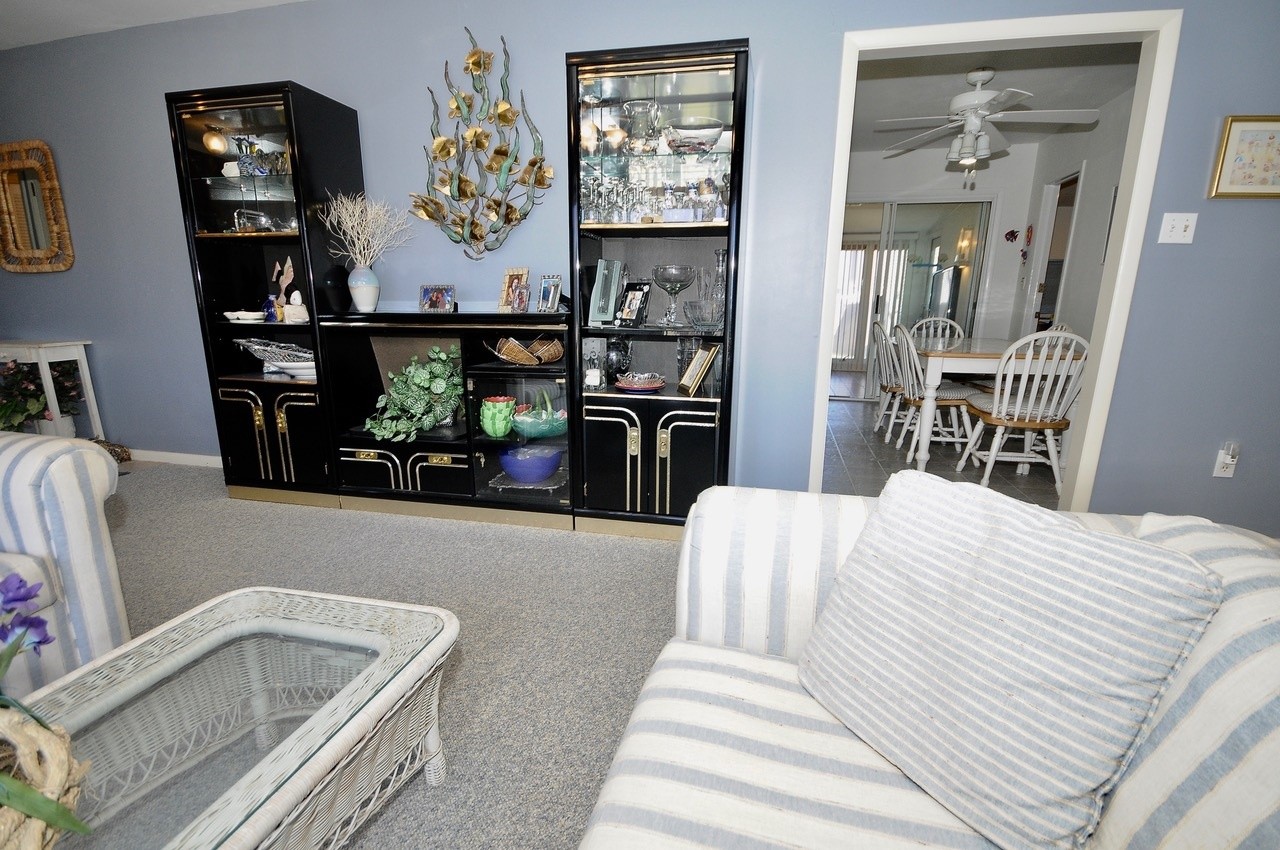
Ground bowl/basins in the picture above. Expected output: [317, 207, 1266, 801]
[617, 372, 659, 386]
[272, 361, 316, 379]
[662, 117, 723, 153]
[682, 298, 725, 331]
[499, 449, 567, 483]
[479, 396, 532, 437]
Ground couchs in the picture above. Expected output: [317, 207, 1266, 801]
[0, 429, 132, 712]
[574, 486, 1280, 849]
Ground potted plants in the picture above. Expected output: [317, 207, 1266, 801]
[362, 347, 464, 441]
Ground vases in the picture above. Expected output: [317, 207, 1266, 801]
[36, 414, 76, 440]
[347, 260, 380, 312]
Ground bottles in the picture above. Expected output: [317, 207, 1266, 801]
[712, 249, 725, 298]
[697, 269, 712, 300]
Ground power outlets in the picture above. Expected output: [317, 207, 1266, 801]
[1213, 449, 1238, 479]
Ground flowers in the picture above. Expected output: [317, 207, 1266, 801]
[0, 572, 90, 850]
[0, 359, 86, 430]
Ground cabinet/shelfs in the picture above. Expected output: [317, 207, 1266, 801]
[314, 312, 574, 512]
[569, 36, 751, 524]
[164, 80, 365, 512]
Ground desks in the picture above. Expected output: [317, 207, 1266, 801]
[889, 336, 1087, 472]
[0, 341, 106, 446]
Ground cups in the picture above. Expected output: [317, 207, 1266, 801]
[658, 192, 716, 222]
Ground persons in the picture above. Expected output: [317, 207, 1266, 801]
[428, 289, 448, 308]
[507, 277, 519, 306]
[626, 293, 638, 317]
[542, 281, 552, 308]
[517, 290, 525, 307]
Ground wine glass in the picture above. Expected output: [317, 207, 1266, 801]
[584, 95, 603, 158]
[652, 265, 696, 327]
[582, 176, 658, 223]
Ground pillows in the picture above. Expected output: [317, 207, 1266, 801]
[798, 468, 1224, 850]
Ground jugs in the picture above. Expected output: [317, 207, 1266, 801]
[621, 101, 662, 156]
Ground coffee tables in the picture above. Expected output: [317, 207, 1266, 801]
[0, 587, 462, 850]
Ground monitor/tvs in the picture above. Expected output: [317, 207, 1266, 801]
[927, 265, 960, 329]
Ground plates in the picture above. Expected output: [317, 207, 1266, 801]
[615, 380, 664, 394]
[223, 312, 267, 318]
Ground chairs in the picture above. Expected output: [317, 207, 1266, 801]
[872, 321, 954, 448]
[911, 317, 964, 386]
[969, 322, 1074, 396]
[957, 330, 1090, 491]
[893, 323, 980, 468]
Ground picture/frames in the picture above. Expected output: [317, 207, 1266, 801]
[539, 274, 562, 312]
[1209, 114, 1280, 200]
[419, 283, 456, 312]
[500, 266, 529, 309]
[613, 282, 651, 329]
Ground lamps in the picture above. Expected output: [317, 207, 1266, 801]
[946, 118, 992, 180]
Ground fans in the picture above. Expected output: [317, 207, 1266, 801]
[874, 68, 1100, 151]
